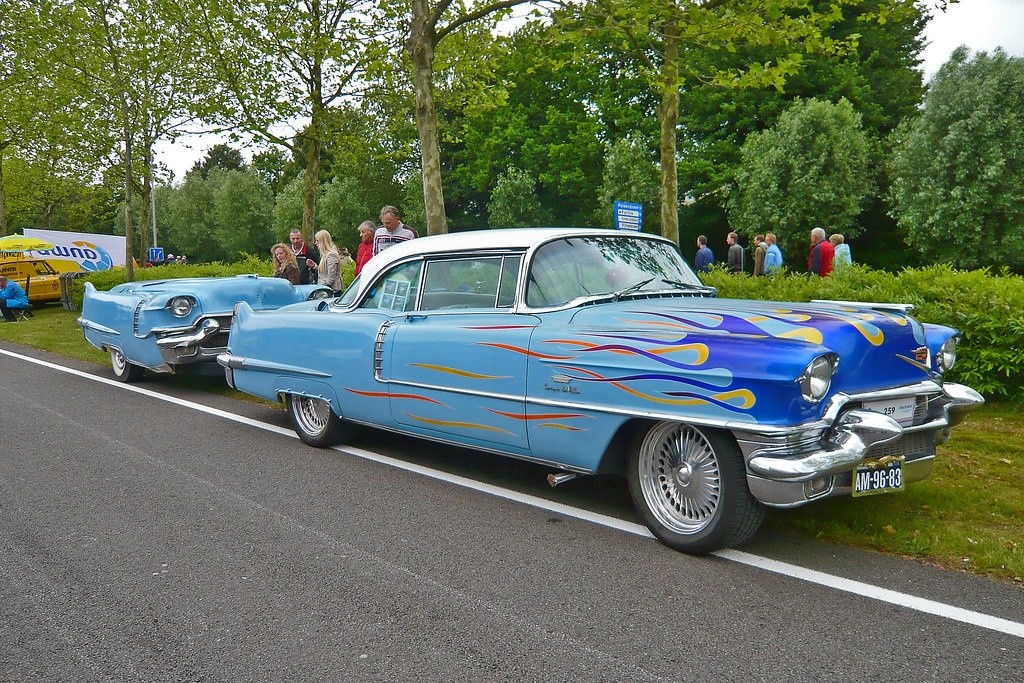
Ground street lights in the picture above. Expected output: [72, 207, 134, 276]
[150, 150, 165, 248]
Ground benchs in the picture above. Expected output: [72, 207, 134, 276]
[404, 291, 508, 311]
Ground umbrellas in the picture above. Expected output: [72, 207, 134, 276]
[0, 233, 55, 286]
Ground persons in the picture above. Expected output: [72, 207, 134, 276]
[271, 244, 301, 285]
[753, 235, 769, 276]
[726, 232, 746, 273]
[164, 254, 188, 266]
[762, 234, 782, 275]
[305, 230, 341, 297]
[829, 234, 851, 268]
[0, 276, 28, 322]
[371, 205, 419, 283]
[287, 230, 319, 284]
[354, 220, 377, 277]
[694, 235, 715, 272]
[808, 228, 834, 278]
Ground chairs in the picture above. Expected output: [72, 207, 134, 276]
[8, 275, 33, 323]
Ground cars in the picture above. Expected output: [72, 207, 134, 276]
[216, 227, 985, 552]
[0, 256, 71, 302]
